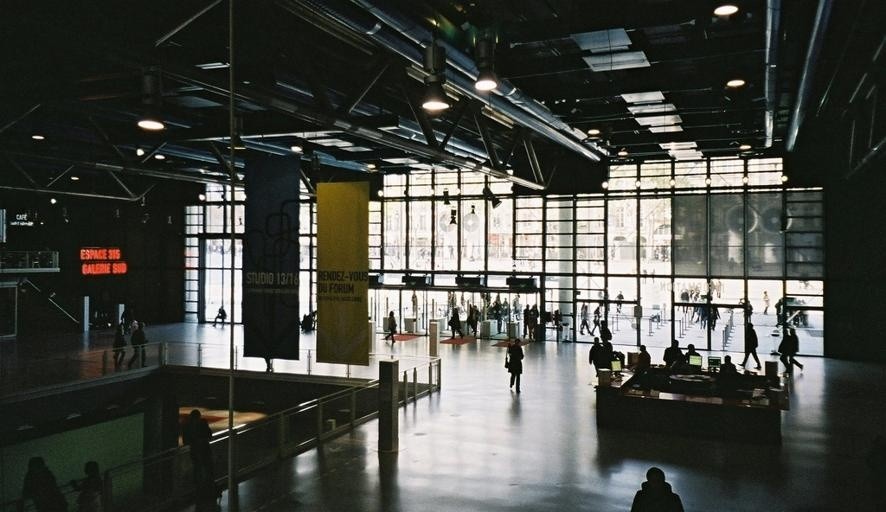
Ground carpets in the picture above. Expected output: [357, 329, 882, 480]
[490, 339, 531, 349]
[439, 335, 474, 345]
[383, 334, 420, 342]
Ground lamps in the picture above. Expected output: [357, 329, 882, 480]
[470, 37, 500, 92]
[438, 180, 503, 228]
[417, 13, 453, 112]
[135, 69, 167, 131]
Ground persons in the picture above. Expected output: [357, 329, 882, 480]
[629, 466, 685, 511]
[411, 290, 418, 315]
[580, 278, 809, 381]
[306, 311, 316, 330]
[22, 456, 68, 512]
[121, 306, 131, 335]
[0, 245, 48, 270]
[213, 306, 226, 326]
[384, 311, 397, 344]
[71, 461, 104, 510]
[182, 409, 218, 511]
[443, 291, 540, 342]
[112, 325, 128, 372]
[507, 339, 523, 394]
[127, 321, 148, 369]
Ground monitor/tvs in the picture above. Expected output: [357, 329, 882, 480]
[708, 356, 721, 370]
[689, 355, 702, 370]
[610, 360, 622, 375]
